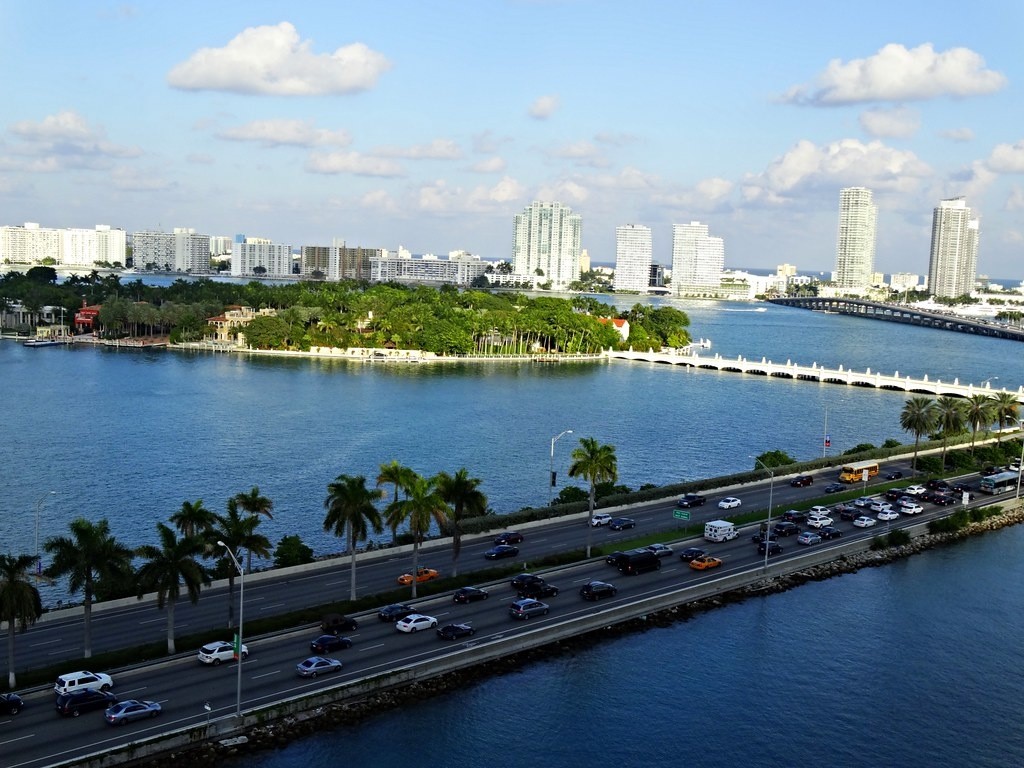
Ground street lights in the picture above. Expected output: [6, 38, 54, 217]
[549, 429, 575, 507]
[1006, 415, 1024, 498]
[218, 540, 243, 715]
[748, 455, 774, 567]
[980, 377, 999, 388]
[36, 491, 57, 592]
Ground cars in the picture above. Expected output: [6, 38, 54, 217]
[678, 495, 706, 508]
[197, 640, 249, 666]
[606, 542, 722, 571]
[294, 528, 558, 679]
[0, 694, 24, 715]
[53, 670, 162, 725]
[717, 496, 742, 510]
[579, 581, 618, 601]
[587, 513, 613, 527]
[610, 518, 635, 531]
[751, 462, 1023, 556]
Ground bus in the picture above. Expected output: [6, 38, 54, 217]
[839, 460, 879, 483]
[980, 471, 1020, 495]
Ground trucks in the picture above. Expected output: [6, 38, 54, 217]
[704, 520, 740, 542]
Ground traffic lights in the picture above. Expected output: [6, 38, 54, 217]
[826, 439, 830, 447]
